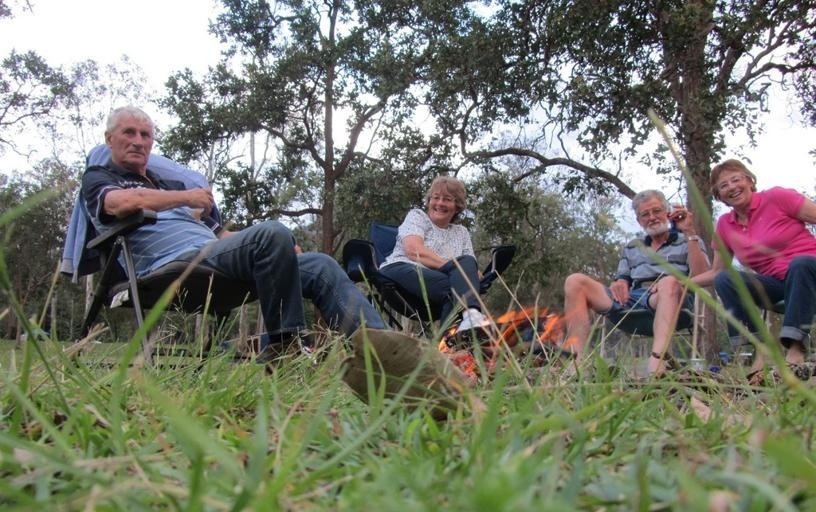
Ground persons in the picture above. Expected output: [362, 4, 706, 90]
[377, 175, 504, 338]
[82, 106, 391, 377]
[562, 189, 712, 385]
[649, 158, 816, 387]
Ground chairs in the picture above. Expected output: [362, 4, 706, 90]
[593, 268, 816, 364]
[343, 221, 517, 343]
[64, 144, 261, 382]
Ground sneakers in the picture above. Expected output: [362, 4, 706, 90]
[454, 319, 491, 333]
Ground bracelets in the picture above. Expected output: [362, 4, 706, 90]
[686, 234, 700, 242]
